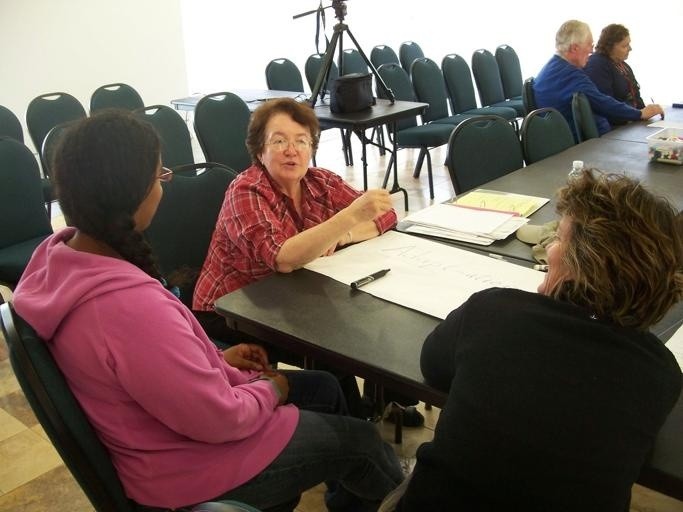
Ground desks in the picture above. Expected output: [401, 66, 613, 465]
[170, 86, 436, 210]
[212, 105, 681, 496]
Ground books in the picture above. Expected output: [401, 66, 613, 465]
[401, 187, 551, 247]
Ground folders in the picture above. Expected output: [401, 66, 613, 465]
[406, 188, 551, 246]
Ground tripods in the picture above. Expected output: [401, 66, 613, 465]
[292, 1, 396, 111]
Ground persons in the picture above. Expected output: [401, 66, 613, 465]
[12, 112, 418, 512]
[191, 100, 423, 426]
[534, 21, 664, 138]
[586, 24, 647, 124]
[371, 172, 681, 511]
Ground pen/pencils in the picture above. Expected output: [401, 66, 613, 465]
[650, 97, 655, 104]
[351, 269, 390, 289]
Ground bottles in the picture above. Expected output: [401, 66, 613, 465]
[569, 160, 584, 180]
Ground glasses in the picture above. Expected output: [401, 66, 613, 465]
[261, 135, 314, 152]
[154, 166, 173, 183]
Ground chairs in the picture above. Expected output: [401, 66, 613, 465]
[372, 45, 399, 70]
[523, 107, 576, 164]
[40, 118, 79, 185]
[336, 48, 390, 153]
[0, 303, 300, 512]
[495, 44, 522, 99]
[132, 105, 196, 175]
[571, 91, 601, 143]
[447, 119, 524, 197]
[27, 92, 88, 222]
[472, 49, 525, 115]
[305, 53, 355, 167]
[399, 41, 425, 77]
[193, 92, 254, 171]
[143, 162, 279, 371]
[410, 57, 483, 180]
[266, 58, 303, 91]
[91, 84, 146, 115]
[443, 53, 518, 140]
[0, 105, 23, 143]
[376, 64, 456, 198]
[0, 138, 54, 302]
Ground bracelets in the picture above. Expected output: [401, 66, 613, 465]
[345, 229, 356, 245]
